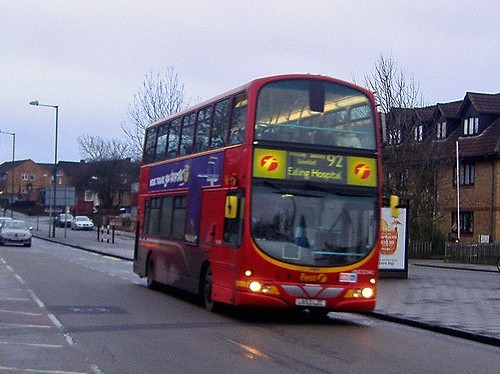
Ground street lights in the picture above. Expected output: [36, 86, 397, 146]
[91, 176, 102, 229]
[30, 100, 59, 238]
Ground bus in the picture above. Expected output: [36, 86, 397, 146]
[133, 74, 401, 316]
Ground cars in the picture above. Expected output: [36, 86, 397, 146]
[0, 217, 12, 229]
[70, 215, 94, 231]
[54, 213, 75, 227]
[0, 220, 33, 247]
